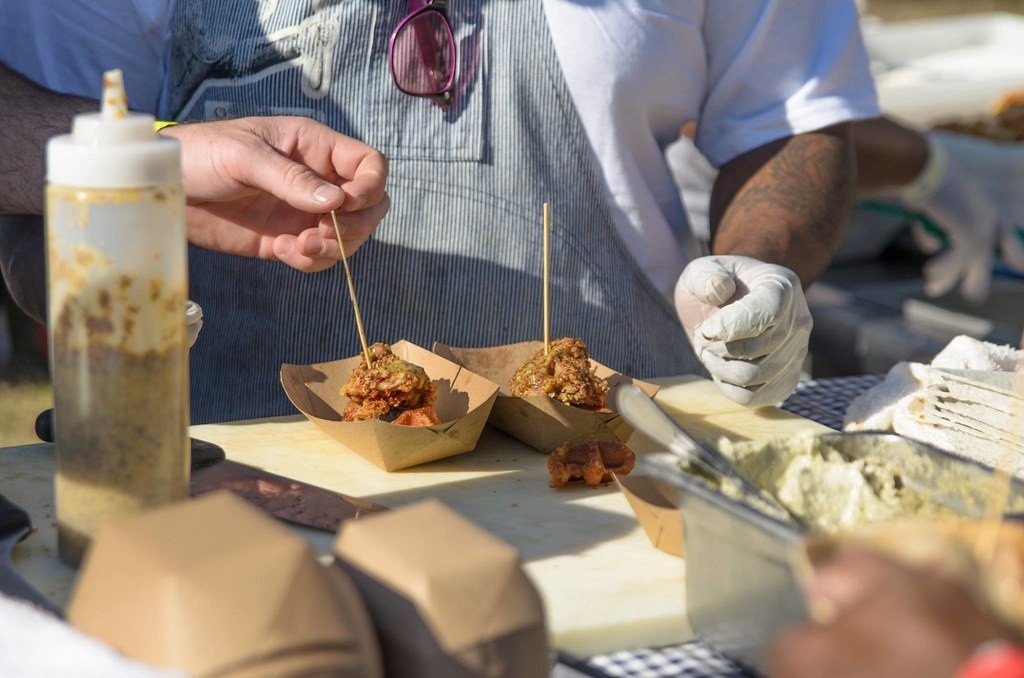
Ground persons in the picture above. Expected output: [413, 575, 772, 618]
[1, 61, 393, 275]
[852, 113, 1024, 310]
[1, 1, 881, 428]
[756, 532, 1024, 677]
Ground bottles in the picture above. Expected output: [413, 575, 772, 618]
[45, 68, 197, 570]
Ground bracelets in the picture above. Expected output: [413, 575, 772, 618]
[155, 121, 179, 132]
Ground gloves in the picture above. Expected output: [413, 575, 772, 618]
[674, 254, 814, 407]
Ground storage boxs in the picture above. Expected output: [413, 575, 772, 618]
[635, 426, 1024, 678]
[279, 338, 503, 472]
[431, 340, 663, 454]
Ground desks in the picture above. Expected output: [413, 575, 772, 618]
[0, 373, 885, 678]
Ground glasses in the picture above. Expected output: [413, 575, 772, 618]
[389, 0, 456, 106]
